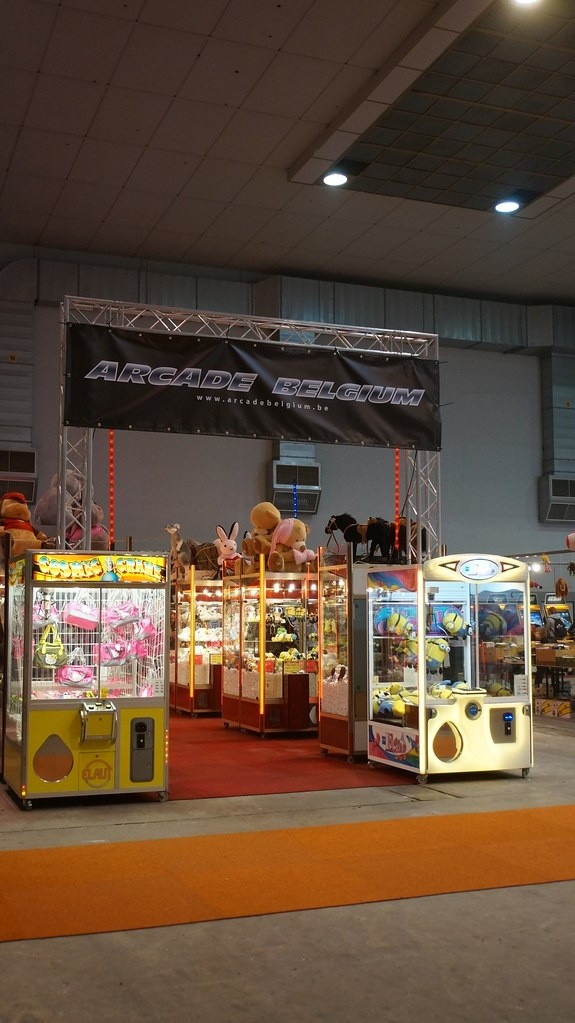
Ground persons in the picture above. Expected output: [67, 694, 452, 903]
[478, 604, 490, 622]
[556, 578, 569, 598]
[532, 626, 548, 687]
[546, 608, 570, 649]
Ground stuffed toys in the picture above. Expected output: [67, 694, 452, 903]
[488, 682, 510, 697]
[166, 502, 425, 578]
[0, 470, 109, 556]
[170, 603, 348, 684]
[481, 612, 507, 636]
[374, 611, 468, 718]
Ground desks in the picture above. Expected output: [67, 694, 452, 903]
[533, 664, 575, 700]
[481, 662, 526, 690]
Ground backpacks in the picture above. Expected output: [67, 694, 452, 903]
[547, 617, 567, 638]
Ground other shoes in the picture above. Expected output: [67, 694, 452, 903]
[534, 683, 542, 688]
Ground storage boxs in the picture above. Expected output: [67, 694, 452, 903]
[478, 646, 511, 664]
[534, 649, 557, 666]
